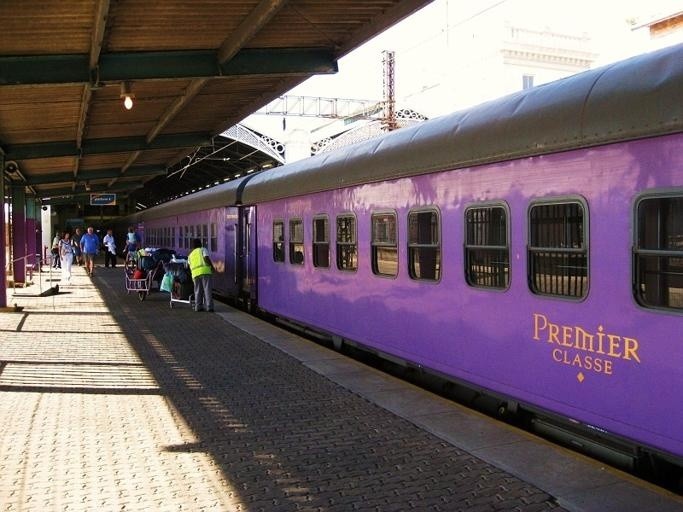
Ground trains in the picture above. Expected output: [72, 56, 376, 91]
[114, 40, 683, 490]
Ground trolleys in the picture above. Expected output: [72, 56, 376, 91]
[168, 258, 195, 310]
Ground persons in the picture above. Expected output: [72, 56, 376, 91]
[57, 232, 75, 285]
[69, 226, 83, 266]
[102, 229, 119, 268]
[123, 224, 143, 264]
[186, 238, 215, 312]
[51, 231, 62, 268]
[79, 226, 99, 274]
[121, 226, 137, 252]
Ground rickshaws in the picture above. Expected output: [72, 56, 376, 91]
[123, 260, 164, 302]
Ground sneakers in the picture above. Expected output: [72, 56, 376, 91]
[207, 309, 213, 312]
[88, 271, 93, 278]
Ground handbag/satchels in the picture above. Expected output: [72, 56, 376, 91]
[117, 248, 127, 259]
[160, 272, 174, 293]
[51, 249, 59, 257]
[72, 246, 80, 256]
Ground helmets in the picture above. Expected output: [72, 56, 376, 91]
[126, 248, 191, 282]
